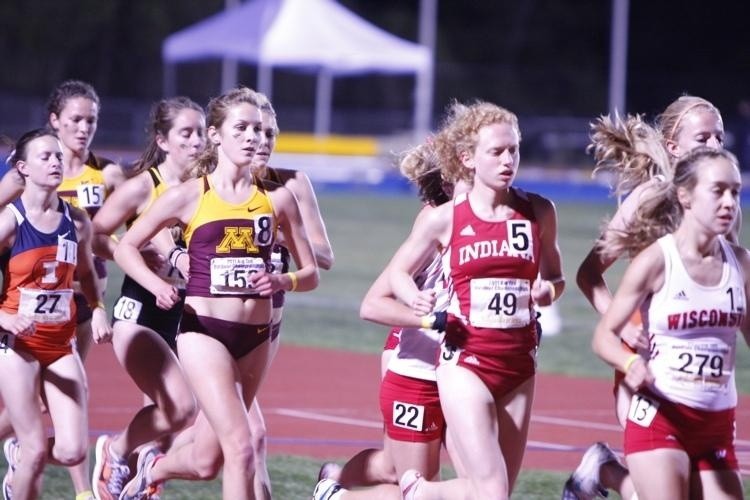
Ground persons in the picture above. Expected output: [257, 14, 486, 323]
[563, 96, 748, 499]
[0, 81, 335, 499]
[313, 97, 567, 499]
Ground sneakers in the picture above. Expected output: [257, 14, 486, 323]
[313, 462, 423, 500]
[92, 434, 165, 500]
[562, 442, 616, 500]
[3, 437, 19, 500]
